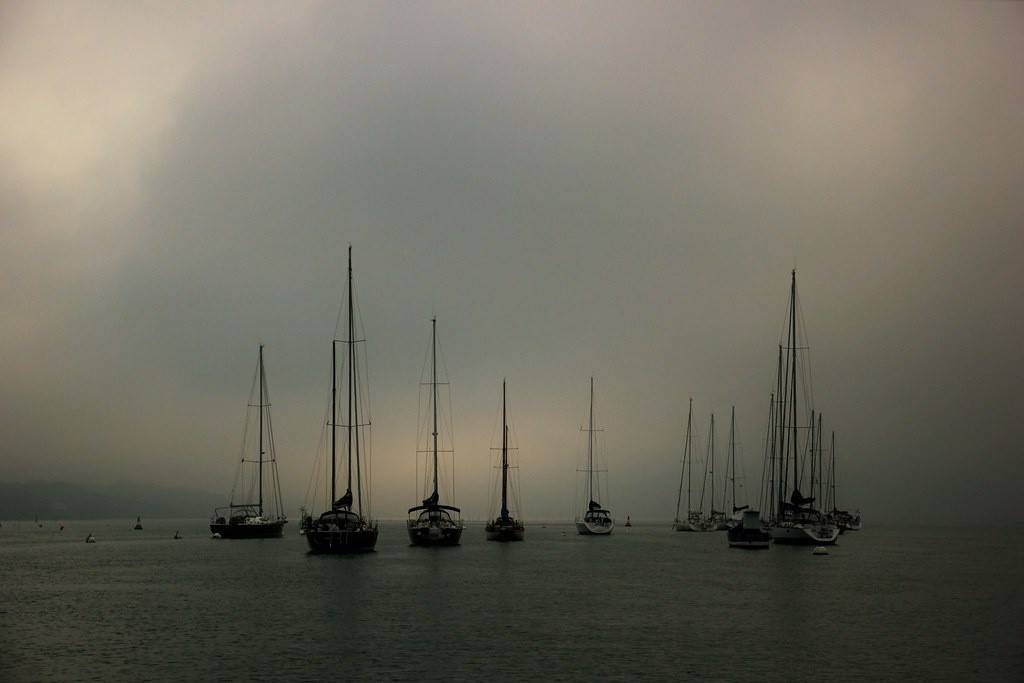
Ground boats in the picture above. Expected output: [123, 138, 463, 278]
[134, 514, 144, 531]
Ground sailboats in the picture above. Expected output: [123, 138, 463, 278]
[574, 376, 617, 535]
[208, 344, 288, 540]
[484, 377, 529, 544]
[299, 245, 381, 559]
[406, 318, 466, 549]
[672, 267, 866, 548]
